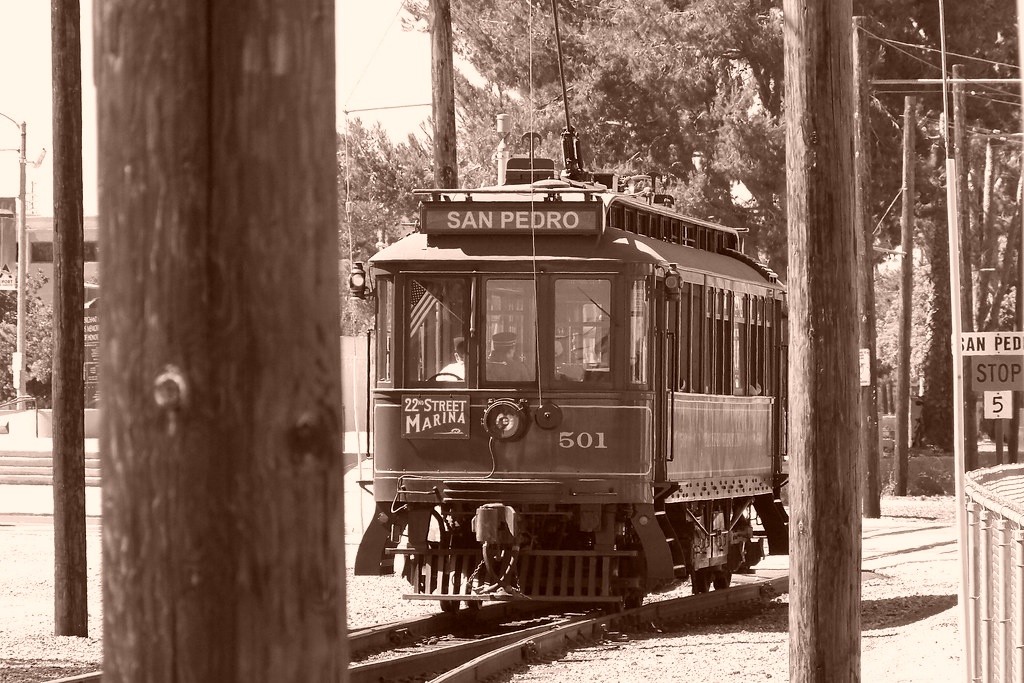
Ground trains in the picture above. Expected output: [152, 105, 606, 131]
[358, 127, 785, 618]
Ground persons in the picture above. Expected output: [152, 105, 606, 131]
[436, 333, 762, 396]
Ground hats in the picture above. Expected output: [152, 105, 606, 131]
[492, 332, 517, 348]
[555, 341, 563, 356]
[454, 336, 466, 356]
